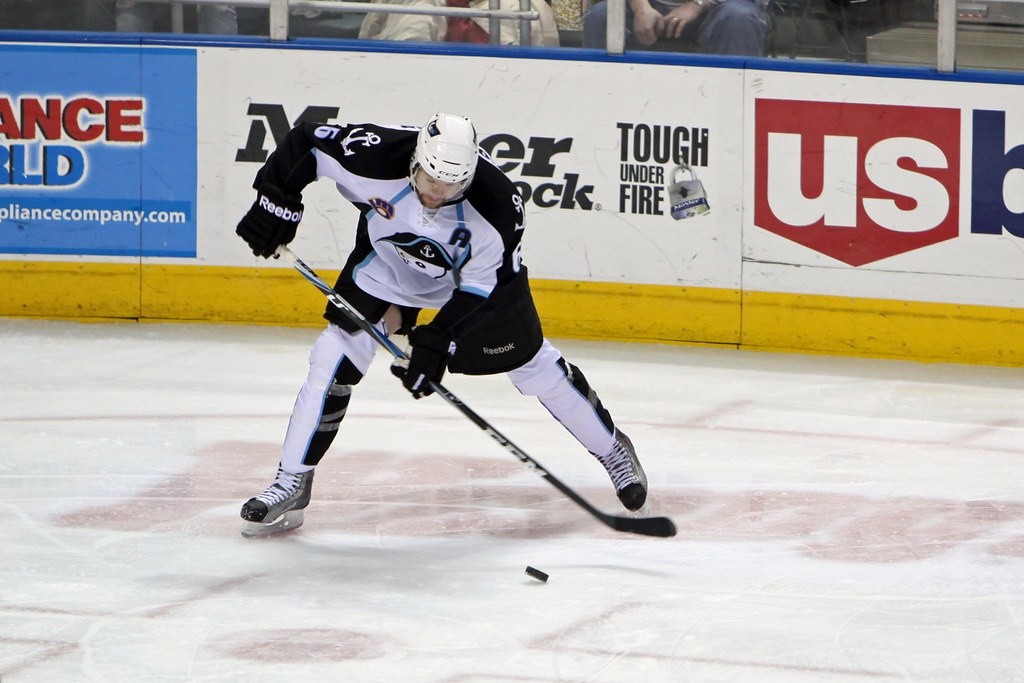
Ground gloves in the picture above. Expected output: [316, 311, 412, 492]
[236, 182, 305, 257]
[390, 308, 460, 399]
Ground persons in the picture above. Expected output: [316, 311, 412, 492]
[358, 0, 562, 47]
[235, 112, 649, 537]
[76, 0, 238, 35]
[582, 0, 773, 56]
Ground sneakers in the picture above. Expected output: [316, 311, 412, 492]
[587, 425, 649, 515]
[237, 461, 315, 536]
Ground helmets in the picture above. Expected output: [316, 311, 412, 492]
[410, 113, 479, 184]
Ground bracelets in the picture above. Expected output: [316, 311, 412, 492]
[694, 0, 704, 8]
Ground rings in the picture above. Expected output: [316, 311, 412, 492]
[673, 17, 678, 22]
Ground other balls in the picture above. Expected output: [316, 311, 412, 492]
[524, 565, 550, 583]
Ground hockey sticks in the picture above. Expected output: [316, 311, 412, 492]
[275, 243, 678, 540]
